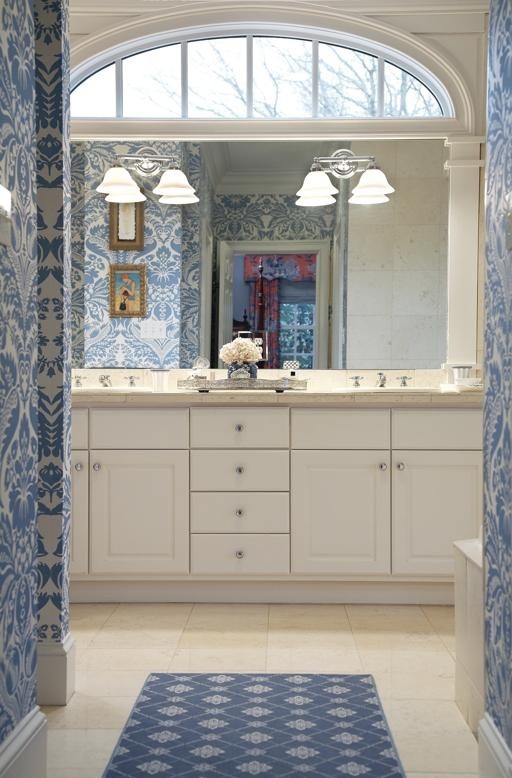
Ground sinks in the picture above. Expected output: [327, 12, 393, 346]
[71, 387, 153, 393]
[334, 386, 430, 392]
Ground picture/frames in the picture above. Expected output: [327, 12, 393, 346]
[108, 263, 147, 318]
[109, 187, 143, 251]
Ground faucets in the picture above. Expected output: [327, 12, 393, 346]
[99, 375, 112, 387]
[377, 373, 387, 386]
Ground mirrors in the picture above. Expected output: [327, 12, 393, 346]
[67, 117, 453, 370]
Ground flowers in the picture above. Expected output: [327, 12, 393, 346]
[219, 337, 263, 366]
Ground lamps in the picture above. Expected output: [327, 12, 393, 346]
[296, 148, 395, 198]
[294, 167, 390, 207]
[104, 163, 200, 206]
[96, 152, 196, 196]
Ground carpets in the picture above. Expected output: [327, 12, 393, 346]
[101, 673, 407, 778]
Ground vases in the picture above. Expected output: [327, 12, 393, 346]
[228, 363, 258, 379]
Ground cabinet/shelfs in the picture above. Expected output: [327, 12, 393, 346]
[288, 407, 487, 584]
[68, 407, 190, 581]
[190, 406, 291, 582]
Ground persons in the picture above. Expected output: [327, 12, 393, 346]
[118, 272, 136, 311]
